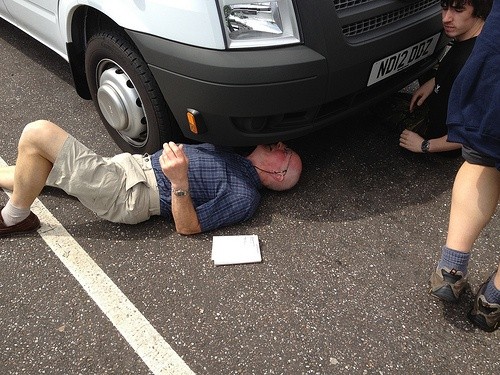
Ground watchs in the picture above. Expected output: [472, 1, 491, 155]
[171, 189, 189, 197]
[421, 138, 433, 154]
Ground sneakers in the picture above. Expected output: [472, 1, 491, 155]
[429, 267, 468, 303]
[467, 269, 500, 332]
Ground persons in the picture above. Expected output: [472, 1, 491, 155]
[0, 120, 302, 234]
[378, 0, 489, 154]
[428, 0, 500, 332]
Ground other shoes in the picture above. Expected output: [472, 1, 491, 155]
[0, 206, 41, 236]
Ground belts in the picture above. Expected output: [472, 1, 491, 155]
[142, 152, 160, 216]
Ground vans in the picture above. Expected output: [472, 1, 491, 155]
[0, 0, 456, 158]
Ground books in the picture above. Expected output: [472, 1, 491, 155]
[211, 235, 262, 265]
